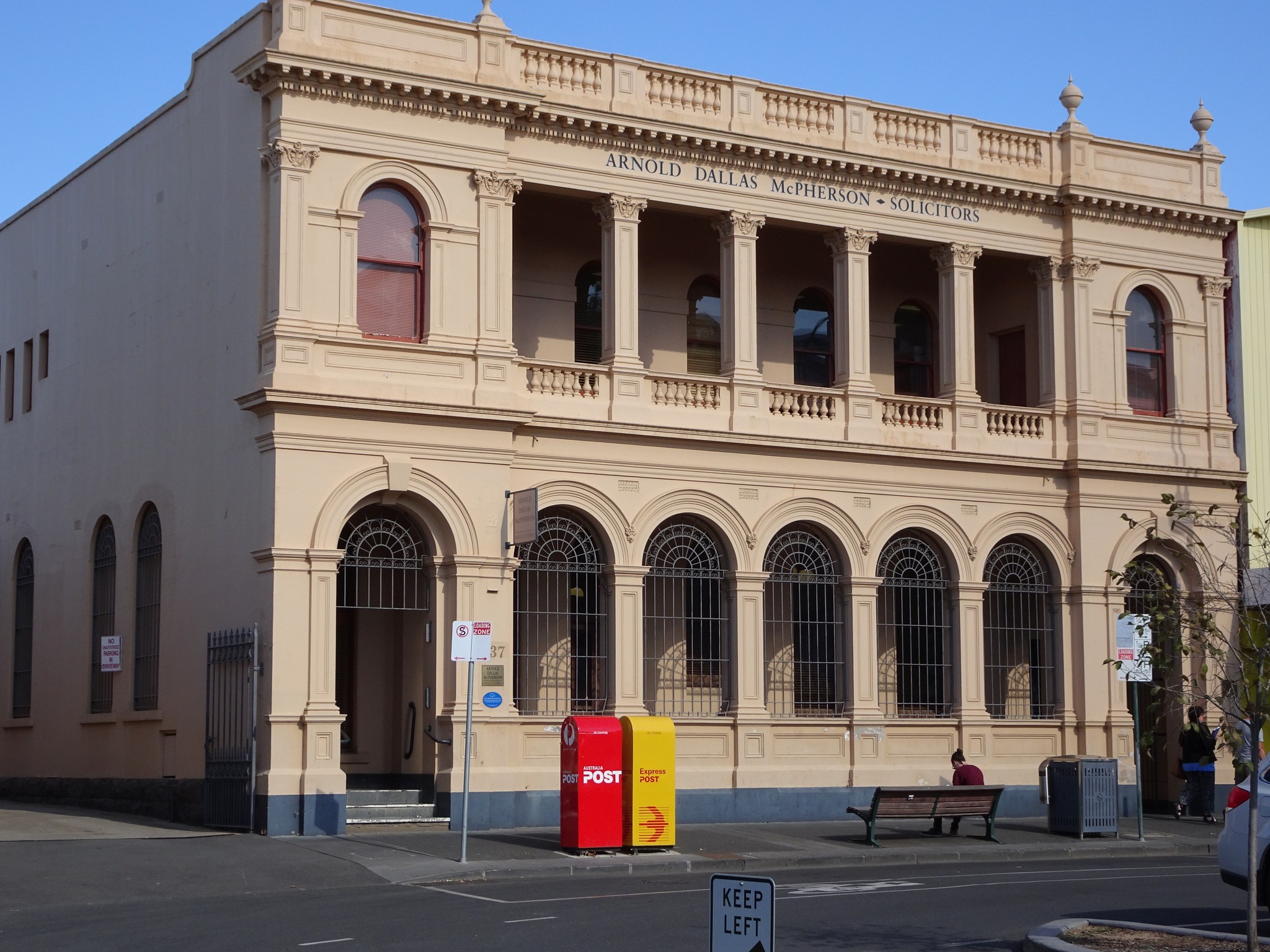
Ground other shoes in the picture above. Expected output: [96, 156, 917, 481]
[1222, 809, 1225, 822]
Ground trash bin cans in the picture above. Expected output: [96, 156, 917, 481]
[1039, 754, 1119, 840]
[560, 716, 623, 856]
[618, 715, 676, 855]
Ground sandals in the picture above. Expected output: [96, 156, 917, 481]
[1204, 814, 1220, 822]
[1173, 802, 1181, 820]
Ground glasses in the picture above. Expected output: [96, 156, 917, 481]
[952, 763, 956, 770]
[1201, 714, 1204, 717]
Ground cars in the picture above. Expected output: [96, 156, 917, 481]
[1216, 750, 1270, 917]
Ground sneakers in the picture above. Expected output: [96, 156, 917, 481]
[949, 828, 958, 836]
[920, 828, 942, 837]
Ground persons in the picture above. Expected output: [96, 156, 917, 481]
[919, 748, 984, 838]
[1172, 706, 1219, 823]
[1232, 703, 1265, 786]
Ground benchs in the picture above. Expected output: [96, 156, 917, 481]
[846, 785, 1005, 846]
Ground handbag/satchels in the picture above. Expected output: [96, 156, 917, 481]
[1175, 758, 1186, 779]
